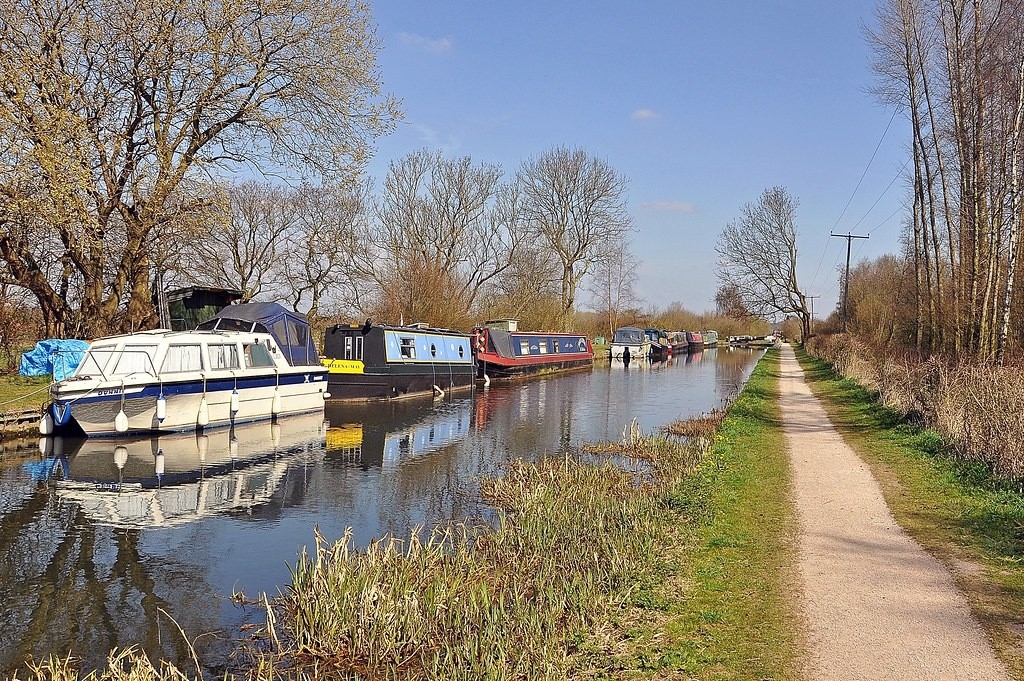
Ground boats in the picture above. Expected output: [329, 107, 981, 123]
[45, 411, 331, 530]
[318, 318, 479, 398]
[50, 329, 332, 437]
[325, 398, 477, 472]
[728, 335, 774, 347]
[609, 325, 719, 358]
[470, 326, 596, 380]
